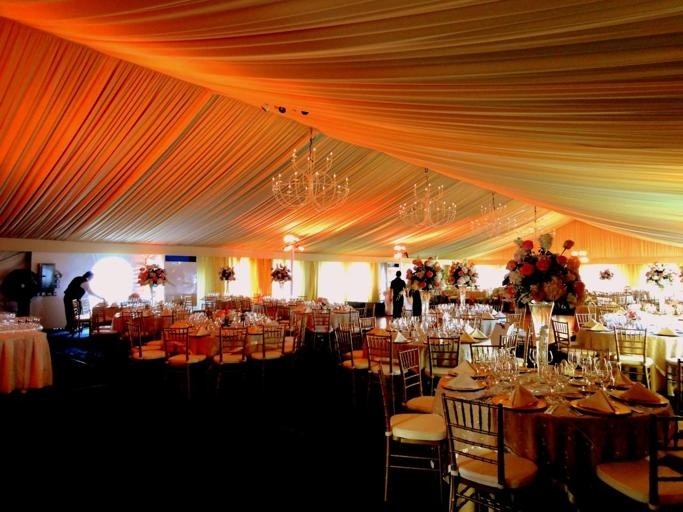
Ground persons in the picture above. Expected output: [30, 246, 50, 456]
[64, 271, 106, 331]
[390, 271, 408, 319]
[409, 289, 422, 323]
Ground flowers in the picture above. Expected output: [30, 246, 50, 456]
[133, 263, 169, 288]
[215, 264, 235, 284]
[443, 258, 480, 289]
[267, 263, 292, 286]
[395, 254, 445, 301]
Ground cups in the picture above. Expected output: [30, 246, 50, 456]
[185, 311, 269, 333]
[0, 310, 41, 333]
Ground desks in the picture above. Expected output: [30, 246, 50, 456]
[1, 323, 53, 395]
[29, 295, 67, 331]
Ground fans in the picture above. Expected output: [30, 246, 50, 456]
[494, 233, 588, 313]
[643, 261, 677, 289]
[597, 268, 614, 281]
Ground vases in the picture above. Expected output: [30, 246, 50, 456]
[457, 285, 467, 308]
[147, 283, 155, 307]
[223, 280, 229, 298]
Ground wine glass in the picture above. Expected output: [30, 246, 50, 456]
[386, 301, 496, 344]
[443, 346, 670, 419]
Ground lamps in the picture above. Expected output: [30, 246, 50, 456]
[395, 162, 456, 230]
[468, 186, 518, 242]
[521, 202, 556, 245]
[265, 121, 353, 214]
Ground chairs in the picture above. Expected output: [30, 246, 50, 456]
[70, 289, 376, 396]
[363, 289, 682, 512]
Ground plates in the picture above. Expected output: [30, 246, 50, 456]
[655, 333, 678, 337]
[581, 324, 611, 332]
